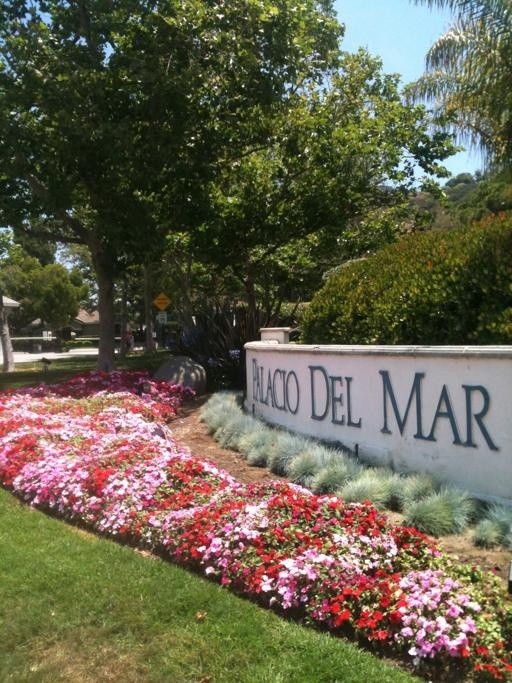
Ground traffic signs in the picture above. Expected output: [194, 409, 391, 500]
[158, 311, 167, 326]
[153, 292, 172, 311]
